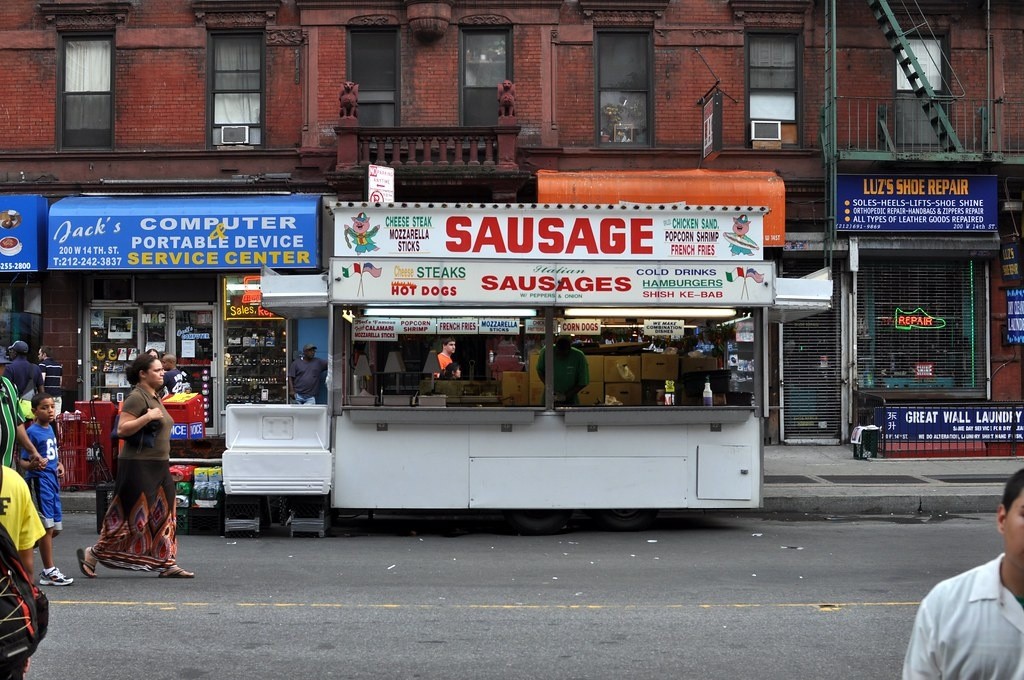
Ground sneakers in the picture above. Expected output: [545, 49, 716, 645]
[40, 568, 73, 585]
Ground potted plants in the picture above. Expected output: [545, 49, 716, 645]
[600, 103, 624, 142]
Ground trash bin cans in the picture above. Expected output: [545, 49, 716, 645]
[852, 427, 878, 459]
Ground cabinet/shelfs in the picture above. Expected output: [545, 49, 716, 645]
[221, 318, 292, 417]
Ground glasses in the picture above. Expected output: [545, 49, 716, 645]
[0, 386, 9, 404]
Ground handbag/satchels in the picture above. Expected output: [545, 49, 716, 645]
[19, 379, 36, 419]
[111, 390, 158, 448]
[0, 522, 49, 680]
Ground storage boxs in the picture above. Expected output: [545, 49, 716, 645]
[96, 405, 331, 538]
[502, 353, 719, 405]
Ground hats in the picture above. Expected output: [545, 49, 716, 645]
[8, 341, 28, 352]
[303, 344, 318, 352]
[0, 349, 13, 364]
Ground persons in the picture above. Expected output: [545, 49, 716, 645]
[147, 349, 159, 358]
[38, 346, 63, 442]
[20, 393, 73, 586]
[288, 344, 328, 405]
[901, 468, 1024, 680]
[0, 345, 47, 472]
[76, 354, 194, 578]
[0, 466, 47, 680]
[2, 340, 45, 430]
[444, 363, 461, 379]
[537, 334, 590, 407]
[433, 336, 456, 378]
[155, 354, 183, 399]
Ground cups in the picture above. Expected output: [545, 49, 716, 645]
[410, 396, 418, 406]
[375, 395, 383, 406]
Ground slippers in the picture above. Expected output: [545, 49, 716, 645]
[158, 569, 195, 578]
[77, 549, 95, 577]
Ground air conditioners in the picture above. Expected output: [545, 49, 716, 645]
[221, 126, 249, 144]
[751, 120, 781, 140]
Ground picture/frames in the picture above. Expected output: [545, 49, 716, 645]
[614, 124, 633, 142]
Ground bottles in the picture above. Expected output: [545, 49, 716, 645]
[90, 309, 287, 429]
[351, 369, 360, 396]
[703, 376, 713, 406]
[488, 350, 494, 363]
[665, 379, 675, 406]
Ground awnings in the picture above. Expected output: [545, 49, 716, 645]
[535, 169, 785, 247]
[46, 195, 322, 270]
